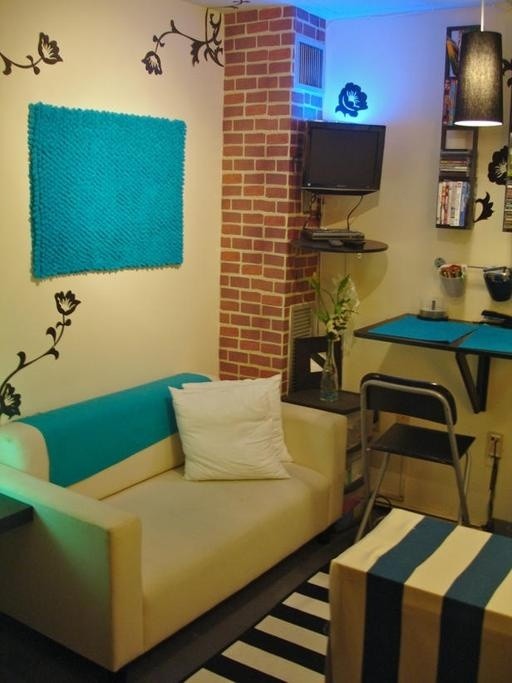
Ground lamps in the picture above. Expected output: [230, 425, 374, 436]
[453, 0, 504, 130]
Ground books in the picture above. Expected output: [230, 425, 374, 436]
[437, 150, 472, 227]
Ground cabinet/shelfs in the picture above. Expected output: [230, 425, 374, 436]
[432, 22, 479, 231]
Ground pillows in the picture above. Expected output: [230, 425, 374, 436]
[166, 371, 295, 481]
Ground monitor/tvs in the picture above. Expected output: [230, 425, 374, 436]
[299, 120, 387, 196]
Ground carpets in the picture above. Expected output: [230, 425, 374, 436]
[170, 557, 333, 682]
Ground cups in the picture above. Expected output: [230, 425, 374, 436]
[482, 268, 512, 302]
[438, 264, 468, 298]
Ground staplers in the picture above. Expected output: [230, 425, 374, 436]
[480, 310, 512, 328]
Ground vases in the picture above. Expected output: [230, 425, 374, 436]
[317, 337, 340, 402]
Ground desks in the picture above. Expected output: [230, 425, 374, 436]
[329, 505, 512, 683]
[352, 312, 511, 415]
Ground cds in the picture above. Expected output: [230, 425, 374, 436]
[419, 306, 447, 319]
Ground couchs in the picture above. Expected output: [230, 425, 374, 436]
[0, 369, 351, 673]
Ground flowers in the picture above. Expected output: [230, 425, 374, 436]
[301, 269, 361, 365]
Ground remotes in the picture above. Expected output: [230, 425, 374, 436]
[328, 239, 344, 246]
[342, 239, 366, 249]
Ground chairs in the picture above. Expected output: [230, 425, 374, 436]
[349, 372, 478, 543]
